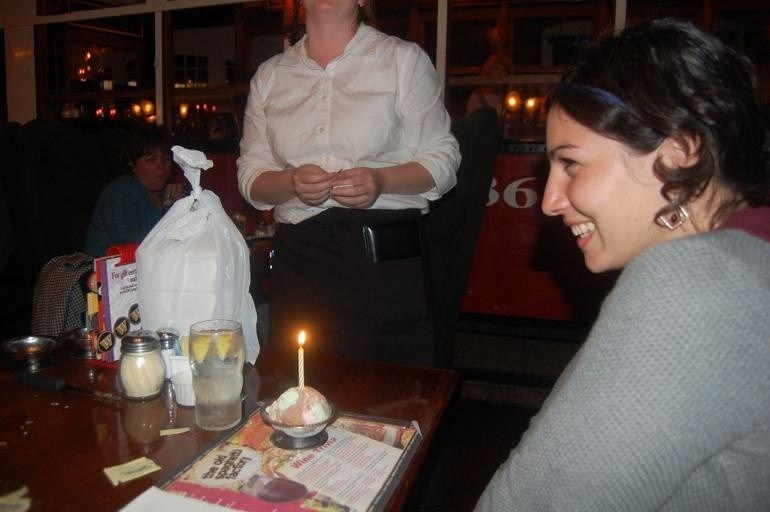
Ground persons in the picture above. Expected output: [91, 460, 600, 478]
[236, 2, 463, 370]
[474, 9, 770, 512]
[79, 132, 190, 258]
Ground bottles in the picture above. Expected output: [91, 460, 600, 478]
[118, 327, 180, 403]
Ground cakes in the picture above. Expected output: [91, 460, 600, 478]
[266, 385, 331, 427]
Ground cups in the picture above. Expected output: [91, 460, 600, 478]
[170, 355, 196, 407]
[189, 319, 245, 431]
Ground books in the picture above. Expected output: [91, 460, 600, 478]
[131, 403, 425, 511]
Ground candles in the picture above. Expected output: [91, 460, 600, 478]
[298, 331, 305, 389]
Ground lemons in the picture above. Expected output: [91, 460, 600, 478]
[191, 329, 214, 364]
[214, 332, 232, 360]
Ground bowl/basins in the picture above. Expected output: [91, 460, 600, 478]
[1, 335, 62, 375]
[61, 326, 96, 360]
